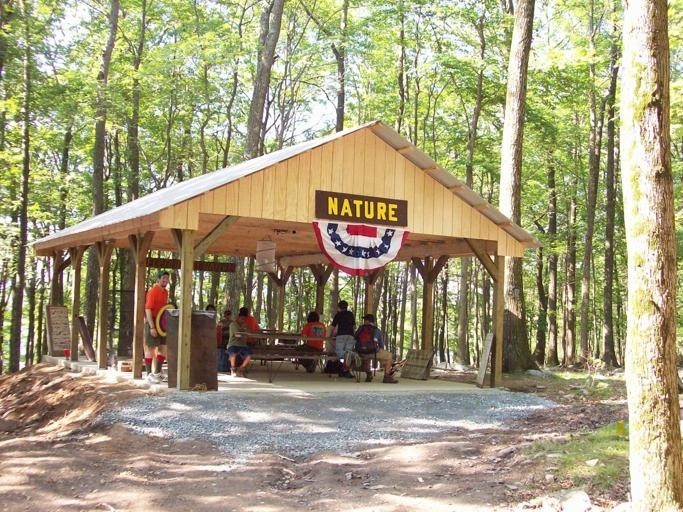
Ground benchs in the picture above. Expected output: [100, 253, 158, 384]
[242, 343, 363, 383]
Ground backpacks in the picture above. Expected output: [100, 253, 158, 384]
[358, 325, 380, 354]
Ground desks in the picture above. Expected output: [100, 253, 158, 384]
[232, 329, 336, 370]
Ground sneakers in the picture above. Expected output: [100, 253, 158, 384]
[148, 373, 168, 384]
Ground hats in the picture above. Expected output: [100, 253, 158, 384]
[361, 314, 374, 320]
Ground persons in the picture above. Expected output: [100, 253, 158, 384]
[294, 311, 325, 374]
[142, 270, 170, 385]
[353, 311, 398, 384]
[206, 303, 262, 377]
[330, 299, 356, 378]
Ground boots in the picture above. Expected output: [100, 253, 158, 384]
[366, 372, 373, 382]
[383, 375, 398, 383]
[239, 368, 247, 378]
[230, 368, 238, 377]
